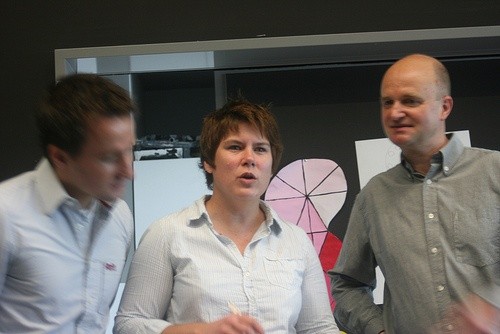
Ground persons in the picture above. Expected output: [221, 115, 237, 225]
[0, 73, 135, 333]
[112, 102, 338, 334]
[328, 54, 500, 334]
